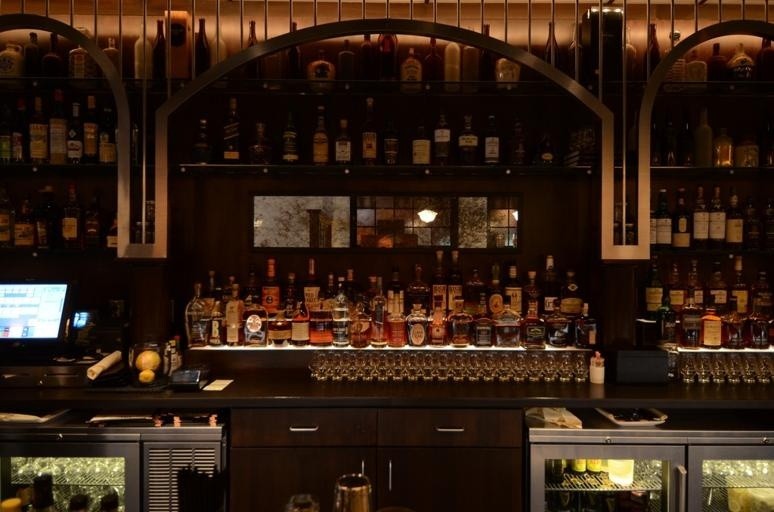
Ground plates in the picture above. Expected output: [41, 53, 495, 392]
[81, 374, 169, 394]
[592, 406, 668, 427]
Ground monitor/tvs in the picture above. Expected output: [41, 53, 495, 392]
[0, 280, 74, 365]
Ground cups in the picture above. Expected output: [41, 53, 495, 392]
[11, 456, 125, 512]
[308, 350, 590, 384]
[679, 355, 774, 385]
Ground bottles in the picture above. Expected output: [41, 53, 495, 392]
[645, 255, 666, 319]
[290, 293, 310, 344]
[407, 304, 429, 347]
[520, 300, 546, 351]
[547, 458, 570, 485]
[0, 44, 22, 82]
[587, 460, 610, 477]
[446, 296, 473, 347]
[524, 271, 543, 320]
[710, 187, 726, 252]
[398, 48, 423, 94]
[408, 265, 432, 316]
[546, 299, 570, 347]
[377, 35, 398, 95]
[284, 274, 298, 320]
[383, 137, 402, 164]
[28, 95, 47, 166]
[686, 49, 708, 94]
[267, 305, 292, 344]
[570, 457, 589, 476]
[668, 264, 687, 313]
[499, 57, 521, 89]
[0, 181, 14, 248]
[224, 277, 236, 302]
[726, 195, 745, 251]
[346, 270, 358, 298]
[42, 30, 64, 78]
[433, 250, 448, 316]
[98, 108, 117, 165]
[358, 31, 376, 94]
[135, 222, 150, 245]
[37, 186, 60, 220]
[284, 20, 303, 95]
[617, 490, 632, 511]
[363, 97, 378, 167]
[311, 104, 328, 166]
[432, 115, 449, 166]
[484, 115, 500, 167]
[761, 124, 774, 168]
[562, 272, 584, 316]
[243, 21, 267, 80]
[384, 286, 409, 349]
[693, 185, 709, 252]
[615, 204, 626, 224]
[261, 257, 280, 314]
[36, 474, 54, 512]
[336, 276, 348, 304]
[575, 304, 597, 349]
[335, 120, 351, 166]
[135, 25, 154, 78]
[631, 490, 650, 511]
[84, 94, 97, 165]
[426, 293, 450, 345]
[243, 296, 268, 347]
[672, 188, 693, 251]
[307, 49, 337, 97]
[174, 335, 183, 358]
[741, 295, 769, 349]
[657, 189, 671, 251]
[702, 485, 710, 511]
[504, 268, 523, 317]
[369, 270, 388, 347]
[26, 503, 35, 512]
[696, 107, 714, 167]
[463, 271, 487, 317]
[543, 256, 562, 317]
[656, 285, 677, 345]
[488, 266, 504, 316]
[666, 32, 687, 93]
[86, 194, 100, 238]
[730, 43, 755, 94]
[462, 48, 480, 91]
[61, 186, 85, 256]
[730, 255, 749, 315]
[15, 482, 30, 511]
[650, 121, 663, 166]
[226, 284, 244, 346]
[209, 288, 225, 347]
[154, 18, 168, 87]
[674, 123, 695, 166]
[714, 128, 732, 167]
[0, 100, 12, 167]
[11, 201, 36, 251]
[204, 271, 216, 310]
[745, 204, 761, 251]
[721, 297, 742, 349]
[756, 36, 774, 94]
[0, 497, 18, 511]
[169, 340, 181, 377]
[426, 36, 446, 92]
[480, 25, 498, 88]
[66, 102, 84, 167]
[492, 304, 521, 346]
[280, 126, 300, 165]
[460, 113, 481, 166]
[70, 493, 87, 511]
[709, 43, 735, 94]
[450, 250, 464, 316]
[385, 271, 403, 315]
[331, 289, 350, 343]
[22, 31, 43, 76]
[647, 23, 662, 80]
[546, 21, 560, 70]
[474, 293, 492, 346]
[289, 493, 319, 511]
[48, 90, 66, 167]
[752, 273, 774, 319]
[223, 98, 241, 164]
[699, 295, 723, 349]
[589, 351, 605, 387]
[705, 262, 728, 309]
[68, 41, 92, 77]
[680, 285, 701, 348]
[762, 195, 774, 251]
[733, 137, 759, 167]
[195, 117, 212, 162]
[100, 492, 120, 511]
[38, 222, 51, 249]
[337, 39, 355, 94]
[504, 116, 530, 166]
[335, 474, 372, 512]
[350, 301, 372, 347]
[248, 122, 275, 166]
[324, 273, 335, 295]
[710, 471, 728, 512]
[614, 224, 622, 244]
[12, 97, 28, 167]
[662, 122, 678, 166]
[447, 42, 461, 92]
[687, 260, 704, 315]
[413, 125, 431, 166]
[650, 187, 656, 251]
[192, 17, 211, 77]
[185, 283, 211, 348]
[304, 256, 323, 313]
[309, 291, 332, 346]
[100, 39, 121, 81]
[628, 223, 638, 245]
[105, 213, 123, 256]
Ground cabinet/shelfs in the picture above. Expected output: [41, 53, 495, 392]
[532, 408, 772, 512]
[0, 415, 225, 512]
[231, 409, 525, 512]
[0, 1, 772, 345]
